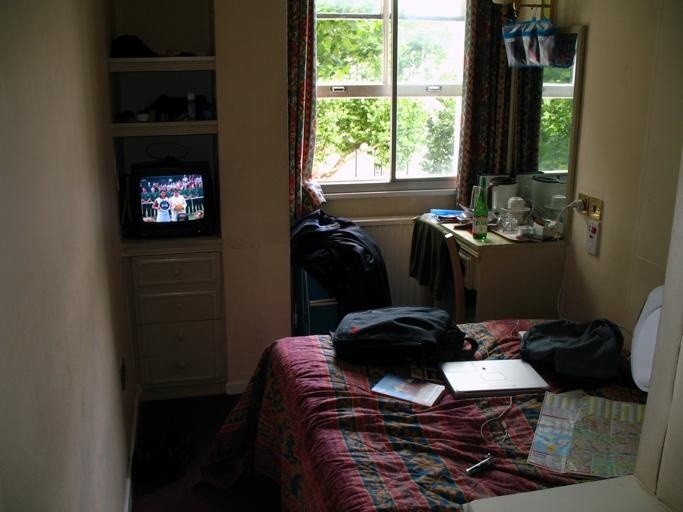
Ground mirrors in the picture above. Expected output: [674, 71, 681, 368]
[507, 25, 584, 241]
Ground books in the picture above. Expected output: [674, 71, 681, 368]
[371, 373, 446, 408]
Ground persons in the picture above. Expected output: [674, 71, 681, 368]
[141, 174, 205, 223]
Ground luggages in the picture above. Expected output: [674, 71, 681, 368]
[294, 264, 338, 336]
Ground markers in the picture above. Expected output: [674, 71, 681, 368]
[466, 458, 492, 476]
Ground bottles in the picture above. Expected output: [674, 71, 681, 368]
[473, 176, 488, 239]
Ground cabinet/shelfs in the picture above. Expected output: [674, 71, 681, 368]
[97, 0, 226, 396]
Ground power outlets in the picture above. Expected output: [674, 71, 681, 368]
[577, 192, 589, 215]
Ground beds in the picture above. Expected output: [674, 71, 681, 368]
[218, 318, 656, 512]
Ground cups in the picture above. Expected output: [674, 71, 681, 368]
[550, 195, 566, 219]
[496, 197, 524, 234]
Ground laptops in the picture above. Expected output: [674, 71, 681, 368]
[438, 358, 552, 399]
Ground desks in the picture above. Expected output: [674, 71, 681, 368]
[433, 212, 565, 321]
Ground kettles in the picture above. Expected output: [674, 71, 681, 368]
[487, 177, 519, 210]
[531, 176, 560, 204]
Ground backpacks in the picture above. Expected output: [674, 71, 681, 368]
[332, 305, 479, 380]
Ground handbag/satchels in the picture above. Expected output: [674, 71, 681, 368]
[520, 318, 624, 389]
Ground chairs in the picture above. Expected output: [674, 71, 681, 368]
[411, 216, 470, 324]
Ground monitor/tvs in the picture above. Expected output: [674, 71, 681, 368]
[131, 159, 219, 241]
